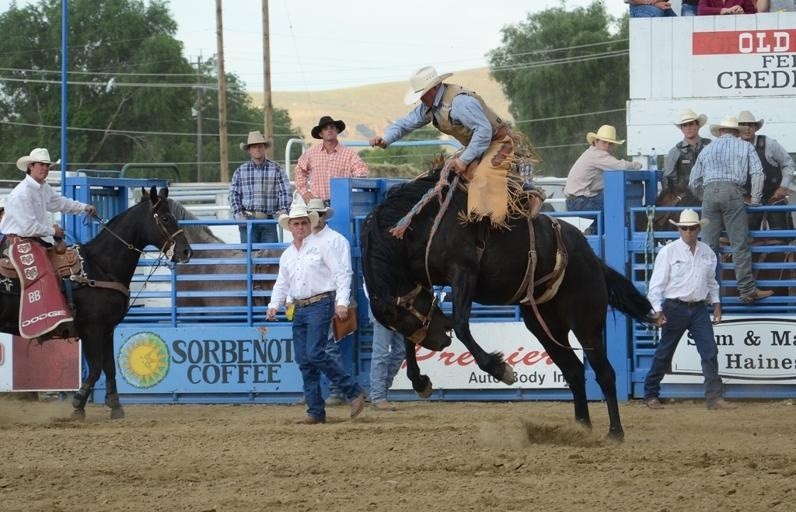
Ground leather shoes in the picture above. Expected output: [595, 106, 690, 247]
[528, 196, 543, 219]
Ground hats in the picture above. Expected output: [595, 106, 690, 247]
[404, 65, 454, 106]
[16, 148, 62, 173]
[586, 108, 765, 228]
[239, 115, 346, 231]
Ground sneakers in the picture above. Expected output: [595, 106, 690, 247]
[743, 289, 774, 303]
[296, 395, 397, 424]
[707, 397, 737, 410]
[647, 397, 665, 409]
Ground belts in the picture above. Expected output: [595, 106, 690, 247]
[293, 295, 329, 306]
[668, 298, 703, 307]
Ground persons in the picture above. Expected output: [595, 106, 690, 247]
[362, 276, 406, 411]
[294, 116, 371, 206]
[228, 131, 294, 252]
[662, 108, 712, 194]
[303, 200, 354, 406]
[643, 209, 738, 409]
[564, 124, 643, 235]
[688, 115, 774, 303]
[266, 206, 366, 424]
[738, 110, 796, 241]
[369, 66, 544, 221]
[0, 147, 96, 338]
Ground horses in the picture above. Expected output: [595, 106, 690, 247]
[0, 185, 193, 422]
[360, 168, 656, 442]
[635, 176, 795, 296]
[141, 186, 285, 306]
